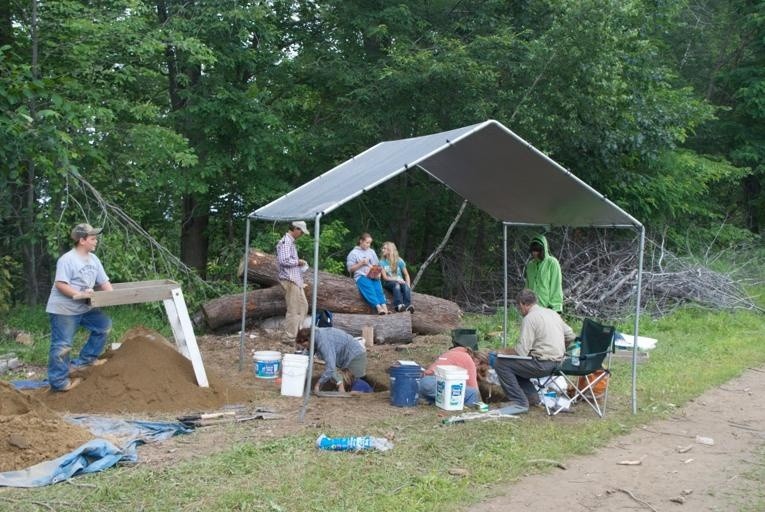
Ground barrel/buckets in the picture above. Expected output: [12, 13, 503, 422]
[385, 364, 421, 408]
[280, 354, 309, 399]
[252, 349, 282, 380]
[436, 364, 469, 410]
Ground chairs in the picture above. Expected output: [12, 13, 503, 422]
[562, 318, 615, 419]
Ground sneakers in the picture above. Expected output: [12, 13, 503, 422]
[500, 404, 529, 415]
[406, 305, 415, 314]
[397, 304, 406, 312]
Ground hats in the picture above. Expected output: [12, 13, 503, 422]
[71, 222, 103, 240]
[293, 220, 310, 235]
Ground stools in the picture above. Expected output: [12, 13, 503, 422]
[532, 353, 575, 415]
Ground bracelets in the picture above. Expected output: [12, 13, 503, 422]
[335, 381, 343, 386]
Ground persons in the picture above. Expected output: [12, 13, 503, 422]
[521, 234, 563, 314]
[296, 327, 368, 394]
[494, 289, 576, 412]
[420, 346, 484, 408]
[45, 224, 113, 392]
[276, 221, 309, 349]
[347, 232, 392, 315]
[380, 241, 415, 314]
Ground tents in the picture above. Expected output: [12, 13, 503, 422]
[238, 119, 645, 416]
